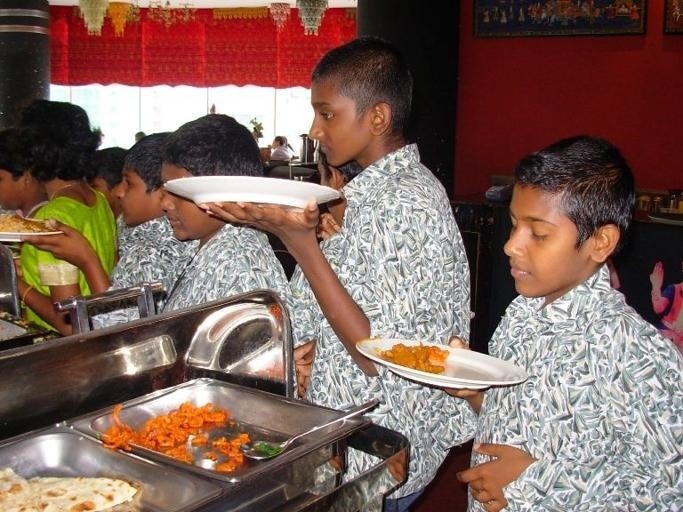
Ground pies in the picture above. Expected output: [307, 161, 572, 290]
[0, 466, 137, 512]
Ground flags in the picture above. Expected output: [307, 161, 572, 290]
[1, 98, 364, 397]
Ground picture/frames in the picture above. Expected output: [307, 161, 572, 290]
[471, 0, 645, 38]
[662, 0, 683, 35]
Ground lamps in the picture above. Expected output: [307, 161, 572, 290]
[79, 0, 329, 36]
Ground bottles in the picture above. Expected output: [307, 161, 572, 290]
[635, 192, 683, 215]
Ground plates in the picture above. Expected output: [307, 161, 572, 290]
[162, 176, 341, 211]
[0, 230, 66, 244]
[355, 337, 529, 390]
[647, 214, 682, 226]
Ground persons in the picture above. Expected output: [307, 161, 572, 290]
[206, 34, 472, 511]
[442, 133, 682, 511]
[649, 260, 683, 351]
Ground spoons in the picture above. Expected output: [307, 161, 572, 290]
[240, 397, 377, 461]
